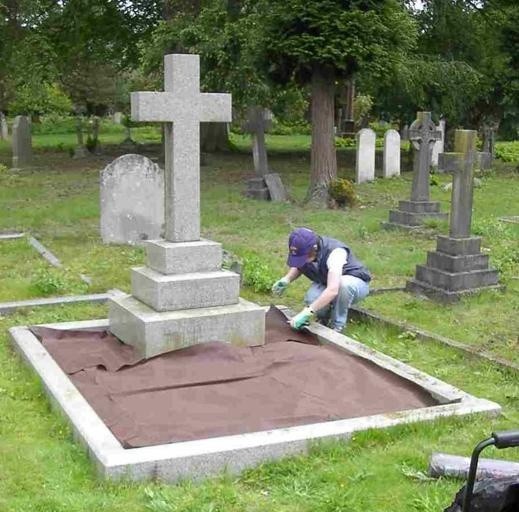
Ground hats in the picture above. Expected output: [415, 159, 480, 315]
[287, 227, 316, 267]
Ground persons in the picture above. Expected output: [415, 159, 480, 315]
[271, 228, 373, 335]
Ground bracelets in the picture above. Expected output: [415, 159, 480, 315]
[306, 306, 316, 317]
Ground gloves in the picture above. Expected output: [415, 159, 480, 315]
[271, 277, 291, 296]
[290, 306, 314, 330]
